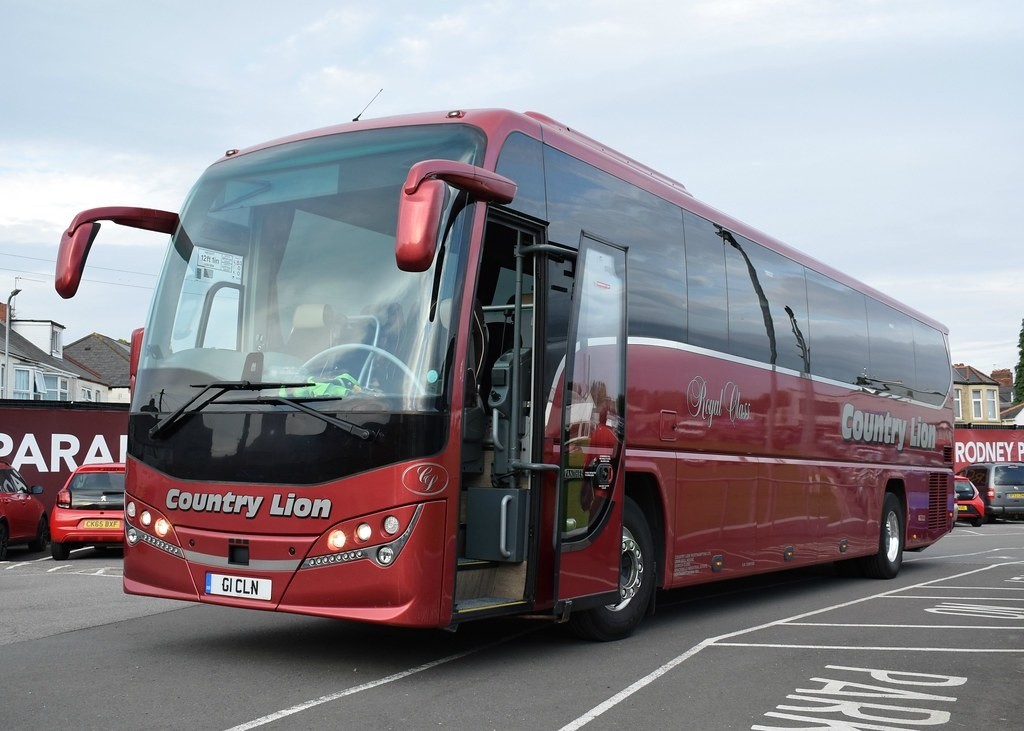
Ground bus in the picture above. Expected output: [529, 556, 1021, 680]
[53, 107, 955, 643]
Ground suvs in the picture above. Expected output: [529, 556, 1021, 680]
[954, 460, 1024, 524]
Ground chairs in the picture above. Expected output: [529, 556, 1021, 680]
[82, 472, 111, 489]
[280, 303, 349, 375]
[346, 301, 405, 391]
[438, 295, 490, 406]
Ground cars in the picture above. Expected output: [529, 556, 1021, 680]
[0, 460, 49, 561]
[954, 474, 984, 527]
[50, 461, 125, 560]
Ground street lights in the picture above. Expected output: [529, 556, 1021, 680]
[4, 288, 21, 399]
